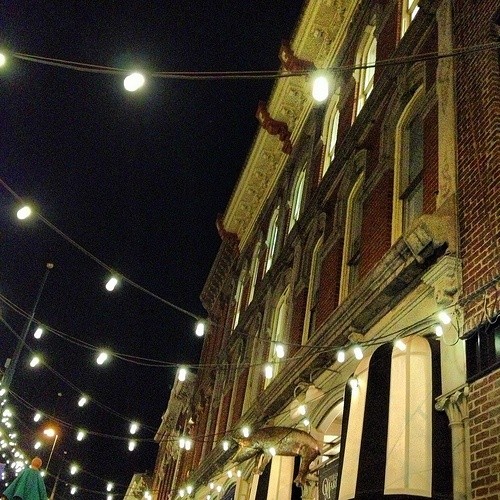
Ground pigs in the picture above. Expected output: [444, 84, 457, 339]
[230, 426, 321, 485]
